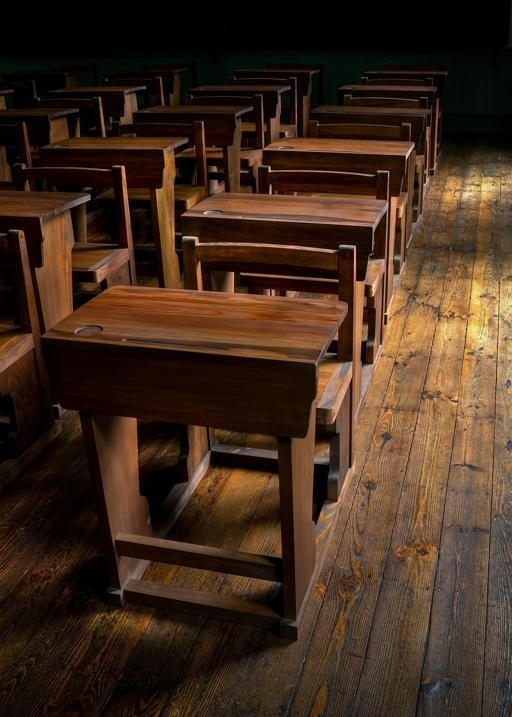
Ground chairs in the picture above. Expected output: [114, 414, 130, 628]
[11, 161, 136, 298]
[186, 91, 264, 193]
[343, 92, 432, 169]
[256, 165, 391, 327]
[111, 117, 208, 289]
[0, 230, 64, 494]
[306, 120, 413, 231]
[179, 236, 364, 503]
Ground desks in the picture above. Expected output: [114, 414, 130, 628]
[0, 188, 93, 421]
[39, 134, 192, 284]
[308, 104, 432, 187]
[341, 83, 437, 102]
[263, 136, 417, 264]
[1, 105, 81, 140]
[230, 66, 326, 137]
[41, 283, 356, 642]
[193, 81, 293, 149]
[48, 84, 149, 131]
[361, 66, 451, 86]
[104, 65, 185, 106]
[133, 104, 254, 189]
[181, 192, 390, 364]
